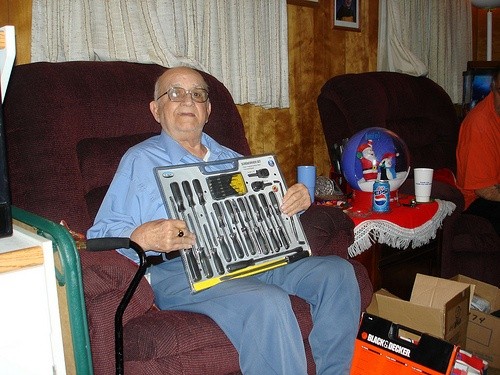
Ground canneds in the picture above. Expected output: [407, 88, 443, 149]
[372, 180, 390, 212]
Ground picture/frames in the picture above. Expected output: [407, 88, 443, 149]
[286, 0, 320, 10]
[330, 0, 361, 32]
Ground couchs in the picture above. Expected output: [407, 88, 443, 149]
[0, 61, 373, 375]
[317, 71, 500, 288]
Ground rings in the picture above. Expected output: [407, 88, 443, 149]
[176, 227, 184, 238]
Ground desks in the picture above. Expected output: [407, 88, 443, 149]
[314, 191, 452, 301]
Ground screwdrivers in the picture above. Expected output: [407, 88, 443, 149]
[153, 155, 313, 293]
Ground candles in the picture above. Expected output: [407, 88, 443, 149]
[487, 9, 492, 61]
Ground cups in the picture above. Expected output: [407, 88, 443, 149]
[413, 167, 434, 203]
[297, 165, 316, 203]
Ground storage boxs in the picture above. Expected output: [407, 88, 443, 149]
[462, 70, 497, 122]
[351, 273, 500, 375]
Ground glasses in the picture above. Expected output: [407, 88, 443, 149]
[156, 87, 209, 103]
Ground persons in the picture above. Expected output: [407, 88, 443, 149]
[356, 138, 382, 182]
[86, 65, 362, 375]
[453, 65, 500, 238]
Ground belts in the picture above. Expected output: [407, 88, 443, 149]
[146, 249, 181, 268]
